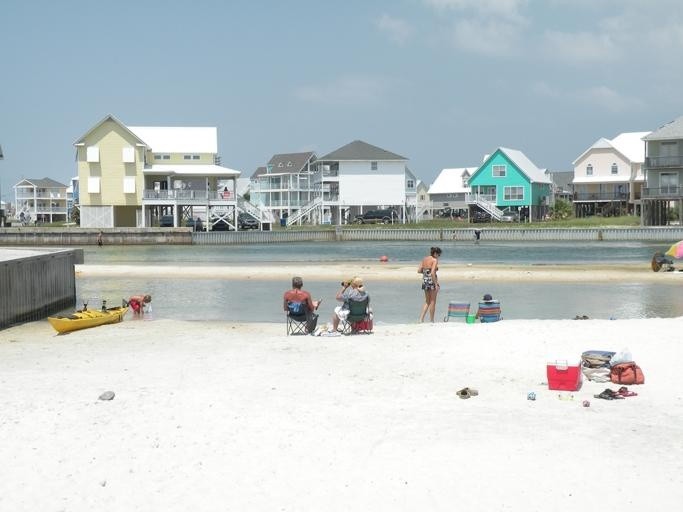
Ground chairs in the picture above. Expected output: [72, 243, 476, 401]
[341, 296, 372, 336]
[282, 300, 315, 336]
[442, 300, 472, 323]
[477, 300, 500, 323]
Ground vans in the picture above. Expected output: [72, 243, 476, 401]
[352, 209, 398, 224]
[209, 211, 230, 231]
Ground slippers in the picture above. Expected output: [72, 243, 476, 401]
[609, 387, 637, 399]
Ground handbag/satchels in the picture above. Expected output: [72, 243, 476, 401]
[610, 361, 644, 384]
[581, 360, 611, 382]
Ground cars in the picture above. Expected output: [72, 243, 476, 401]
[200, 220, 211, 230]
[158, 215, 172, 227]
[438, 207, 465, 217]
[470, 210, 490, 223]
[185, 216, 202, 232]
[500, 211, 523, 223]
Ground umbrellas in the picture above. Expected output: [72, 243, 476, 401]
[666, 240, 683, 262]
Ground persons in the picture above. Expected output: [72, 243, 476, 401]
[473, 229, 483, 240]
[329, 277, 373, 335]
[282, 277, 321, 335]
[475, 295, 493, 321]
[223, 187, 229, 191]
[129, 294, 152, 316]
[419, 246, 444, 321]
[97, 230, 105, 245]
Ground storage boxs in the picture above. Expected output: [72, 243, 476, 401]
[542, 352, 582, 391]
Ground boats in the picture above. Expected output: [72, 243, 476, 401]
[46, 306, 129, 333]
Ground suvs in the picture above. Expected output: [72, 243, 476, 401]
[237, 213, 258, 229]
